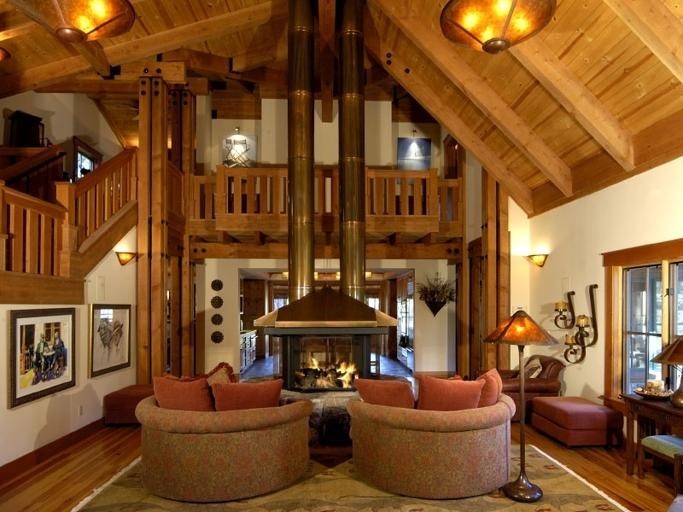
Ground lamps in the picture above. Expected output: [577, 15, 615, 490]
[553, 284, 598, 365]
[650, 336, 683, 408]
[116, 253, 136, 265]
[526, 253, 547, 266]
[24, 1, 137, 43]
[403, 128, 424, 160]
[483, 310, 566, 502]
[441, 1, 555, 53]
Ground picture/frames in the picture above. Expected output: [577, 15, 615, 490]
[88, 303, 130, 377]
[9, 308, 76, 409]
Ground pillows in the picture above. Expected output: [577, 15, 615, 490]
[353, 379, 415, 408]
[516, 358, 542, 379]
[180, 362, 234, 388]
[212, 379, 284, 411]
[152, 376, 213, 411]
[417, 374, 485, 410]
[478, 368, 503, 406]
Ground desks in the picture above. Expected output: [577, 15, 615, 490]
[621, 394, 682, 495]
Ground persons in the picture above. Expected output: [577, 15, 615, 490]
[36, 331, 65, 369]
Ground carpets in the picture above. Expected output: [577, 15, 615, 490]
[69, 435, 629, 512]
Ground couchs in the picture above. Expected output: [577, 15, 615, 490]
[348, 393, 514, 499]
[481, 356, 563, 422]
[134, 394, 312, 503]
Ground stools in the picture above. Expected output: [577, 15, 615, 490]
[103, 382, 152, 426]
[530, 395, 616, 447]
[637, 434, 683, 498]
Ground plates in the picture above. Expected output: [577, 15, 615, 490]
[633, 387, 674, 398]
